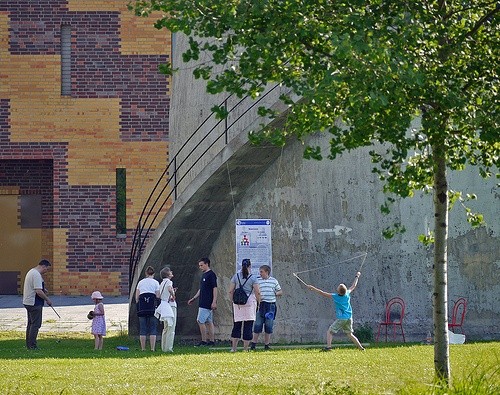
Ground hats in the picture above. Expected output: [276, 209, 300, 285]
[91, 291, 103, 300]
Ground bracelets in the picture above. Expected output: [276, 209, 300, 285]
[355, 275, 359, 278]
[192, 297, 195, 300]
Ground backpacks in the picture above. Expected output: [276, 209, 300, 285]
[232, 272, 254, 305]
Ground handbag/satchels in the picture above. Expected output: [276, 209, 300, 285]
[154, 298, 170, 320]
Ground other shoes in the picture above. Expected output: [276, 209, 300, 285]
[194, 340, 216, 347]
[248, 347, 255, 352]
[322, 346, 331, 351]
[264, 347, 273, 351]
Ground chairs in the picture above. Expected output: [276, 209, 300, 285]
[448, 298, 467, 345]
[377, 297, 405, 347]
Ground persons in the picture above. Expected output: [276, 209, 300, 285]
[136, 266, 160, 352]
[229, 258, 260, 352]
[22, 259, 52, 351]
[188, 257, 217, 349]
[159, 267, 177, 353]
[90, 291, 106, 351]
[306, 272, 365, 352]
[250, 265, 283, 351]
